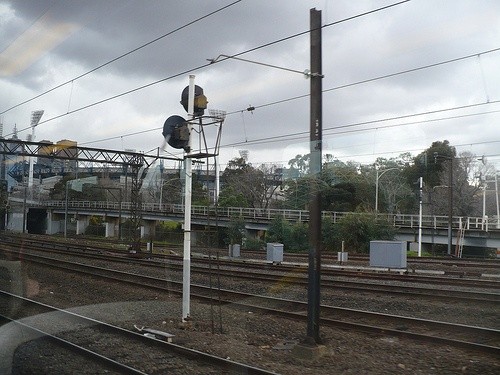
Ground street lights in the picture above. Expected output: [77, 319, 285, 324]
[373, 166, 399, 225]
